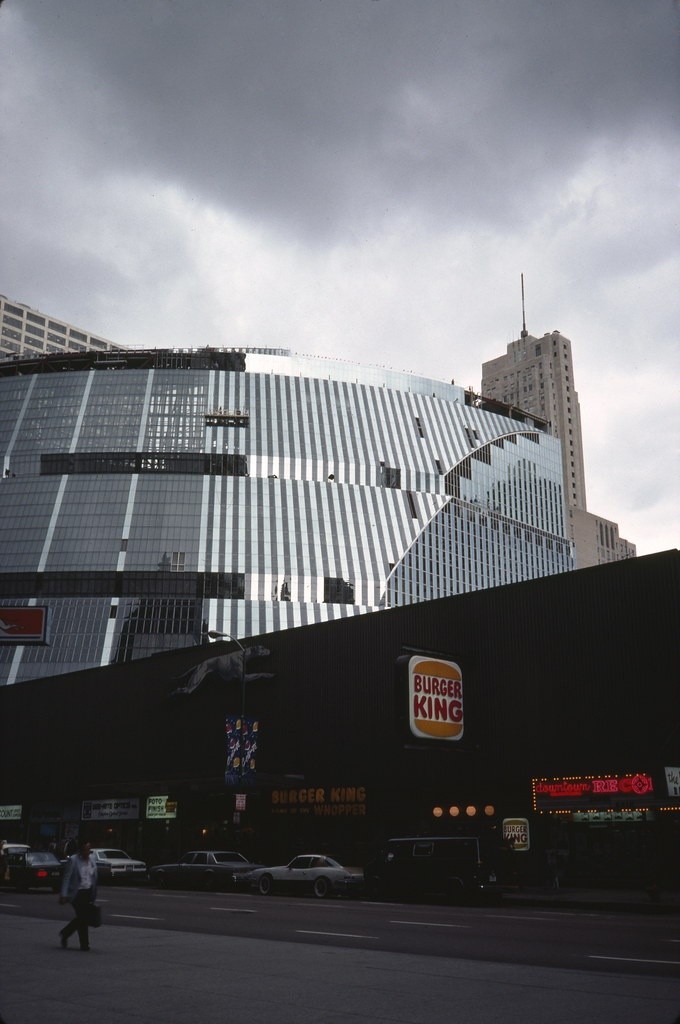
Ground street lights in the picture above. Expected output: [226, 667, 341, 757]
[207, 630, 246, 838]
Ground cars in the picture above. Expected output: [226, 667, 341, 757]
[63, 849, 147, 880]
[252, 854, 359, 898]
[148, 850, 258, 890]
[2, 844, 63, 888]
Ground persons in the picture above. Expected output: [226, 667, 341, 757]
[0, 840, 8, 881]
[59, 842, 98, 951]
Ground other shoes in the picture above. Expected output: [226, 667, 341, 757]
[58, 929, 68, 948]
[80, 944, 89, 951]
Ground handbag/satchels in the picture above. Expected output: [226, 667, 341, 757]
[88, 899, 101, 928]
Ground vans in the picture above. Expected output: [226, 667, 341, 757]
[363, 833, 501, 906]
